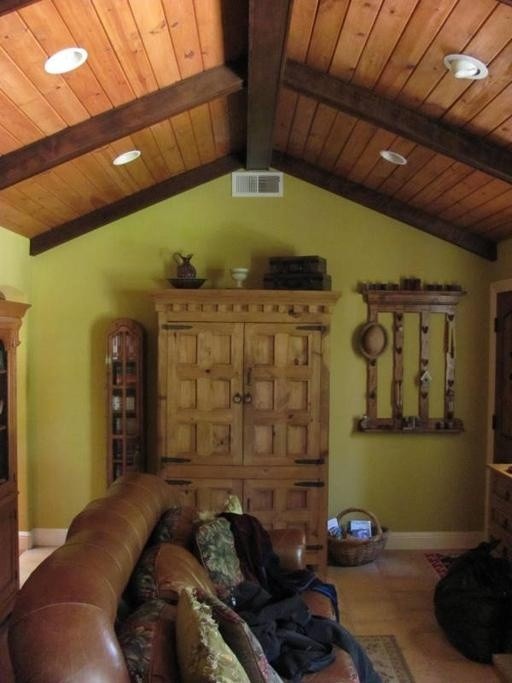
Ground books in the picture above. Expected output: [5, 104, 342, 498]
[113, 332, 136, 484]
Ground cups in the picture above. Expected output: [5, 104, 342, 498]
[439, 421, 445, 429]
[360, 276, 462, 292]
[449, 421, 454, 429]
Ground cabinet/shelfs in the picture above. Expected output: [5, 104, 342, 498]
[1, 490, 25, 625]
[481, 458, 510, 555]
[159, 467, 331, 569]
[1, 292, 33, 498]
[148, 286, 343, 466]
[103, 314, 148, 486]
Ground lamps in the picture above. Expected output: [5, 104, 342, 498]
[447, 54, 480, 79]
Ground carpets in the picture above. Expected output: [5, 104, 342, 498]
[421, 548, 461, 580]
[354, 630, 416, 683]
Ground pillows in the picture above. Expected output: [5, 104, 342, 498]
[195, 581, 285, 683]
[195, 494, 253, 608]
[173, 581, 253, 683]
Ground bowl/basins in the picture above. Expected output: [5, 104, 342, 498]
[167, 277, 208, 289]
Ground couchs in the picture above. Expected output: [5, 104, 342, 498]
[9, 471, 364, 683]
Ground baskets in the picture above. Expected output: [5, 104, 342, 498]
[326, 505, 390, 568]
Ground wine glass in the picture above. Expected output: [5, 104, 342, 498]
[229, 267, 250, 289]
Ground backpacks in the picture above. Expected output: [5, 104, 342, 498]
[433, 537, 512, 664]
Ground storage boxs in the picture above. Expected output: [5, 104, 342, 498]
[268, 253, 328, 273]
[263, 272, 332, 290]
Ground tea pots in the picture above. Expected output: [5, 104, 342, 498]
[173, 250, 197, 280]
